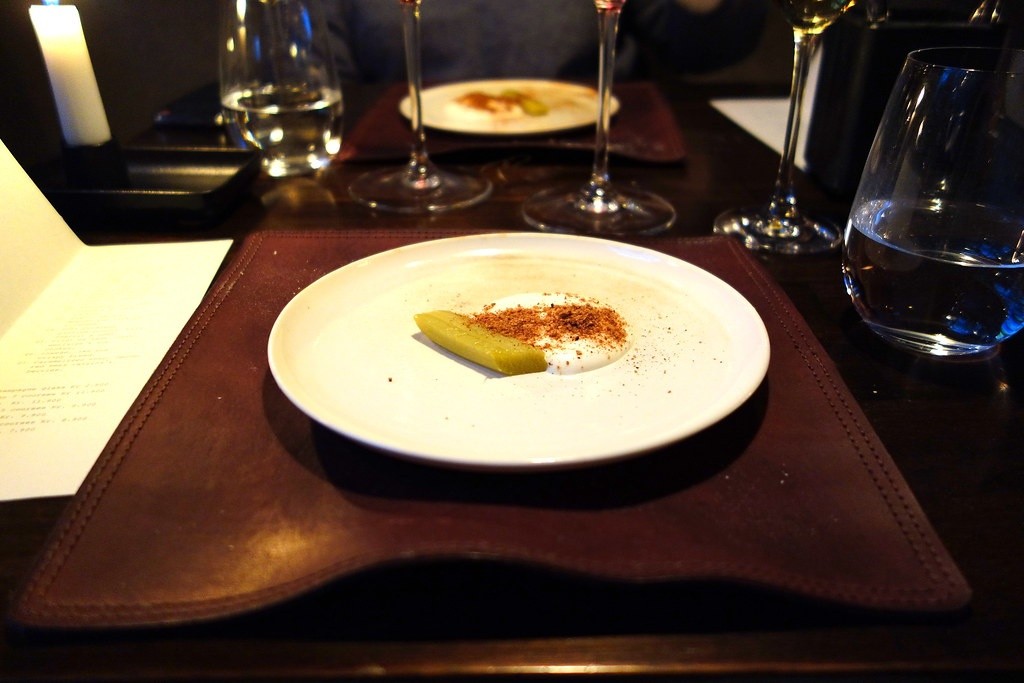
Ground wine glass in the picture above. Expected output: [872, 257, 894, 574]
[523, 1, 677, 238]
[349, 1, 493, 213]
[713, 1, 858, 253]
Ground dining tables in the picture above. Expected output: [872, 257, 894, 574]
[0, 79, 1024, 683]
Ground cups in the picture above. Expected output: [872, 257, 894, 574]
[843, 46, 1024, 355]
[217, 1, 345, 178]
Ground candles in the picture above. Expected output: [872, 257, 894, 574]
[28, 0, 111, 147]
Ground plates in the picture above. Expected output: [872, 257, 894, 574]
[268, 232, 770, 466]
[400, 79, 621, 134]
